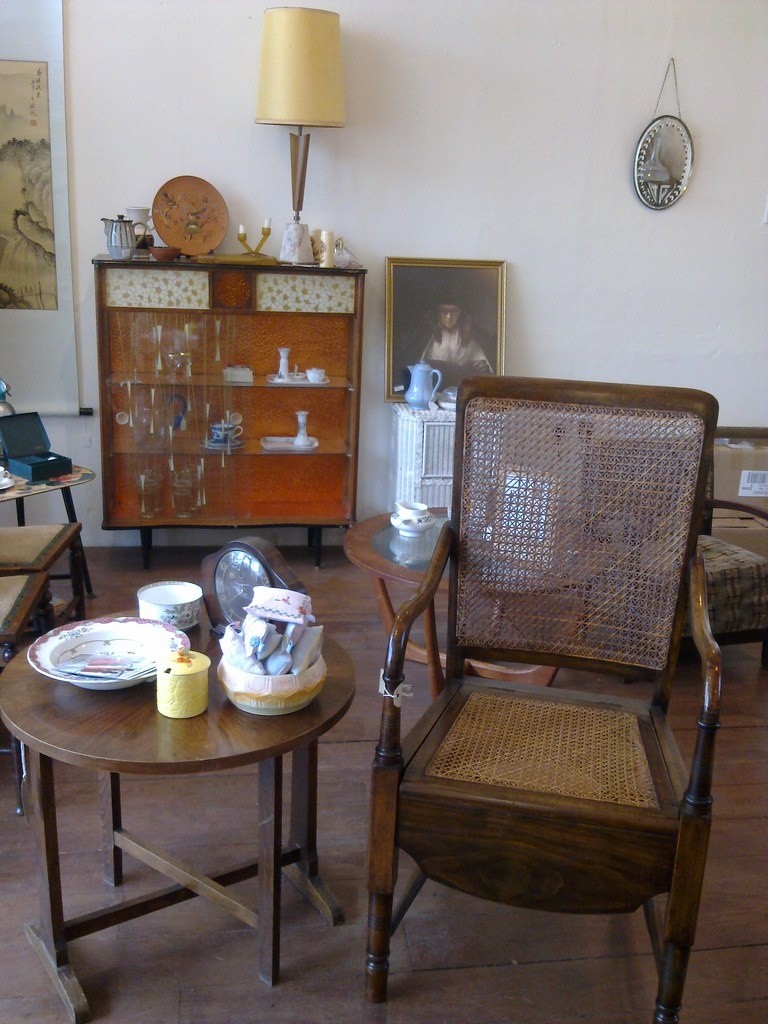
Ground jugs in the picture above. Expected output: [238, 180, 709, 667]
[101, 215, 146, 259]
[389, 502, 435, 537]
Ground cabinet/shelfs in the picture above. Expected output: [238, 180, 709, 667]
[92, 252, 367, 573]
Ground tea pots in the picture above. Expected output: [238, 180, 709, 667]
[404, 361, 442, 410]
[124, 207, 155, 258]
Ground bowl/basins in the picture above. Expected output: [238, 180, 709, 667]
[137, 581, 203, 631]
[148, 247, 181, 262]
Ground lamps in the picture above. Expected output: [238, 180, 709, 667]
[254, 6, 345, 264]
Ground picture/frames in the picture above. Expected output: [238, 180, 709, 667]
[383, 255, 507, 406]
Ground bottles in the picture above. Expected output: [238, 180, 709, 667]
[274, 348, 292, 382]
[294, 412, 311, 445]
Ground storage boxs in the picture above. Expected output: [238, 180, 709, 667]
[0, 411, 72, 481]
[709, 444, 768, 563]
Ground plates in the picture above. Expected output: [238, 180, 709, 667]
[439, 403, 456, 412]
[0, 480, 14, 489]
[27, 617, 191, 690]
[198, 436, 245, 450]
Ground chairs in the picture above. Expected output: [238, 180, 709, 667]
[586, 434, 768, 684]
[363, 374, 723, 1023]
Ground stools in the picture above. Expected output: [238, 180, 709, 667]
[0, 522, 83, 815]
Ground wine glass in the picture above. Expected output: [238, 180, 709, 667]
[165, 350, 194, 382]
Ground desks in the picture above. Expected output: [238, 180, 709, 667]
[0, 465, 96, 596]
[1, 599, 356, 1024]
[346, 505, 605, 685]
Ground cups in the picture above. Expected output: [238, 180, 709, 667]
[136, 464, 203, 520]
[155, 646, 211, 718]
[306, 370, 325, 382]
[0, 467, 10, 484]
[210, 422, 243, 445]
